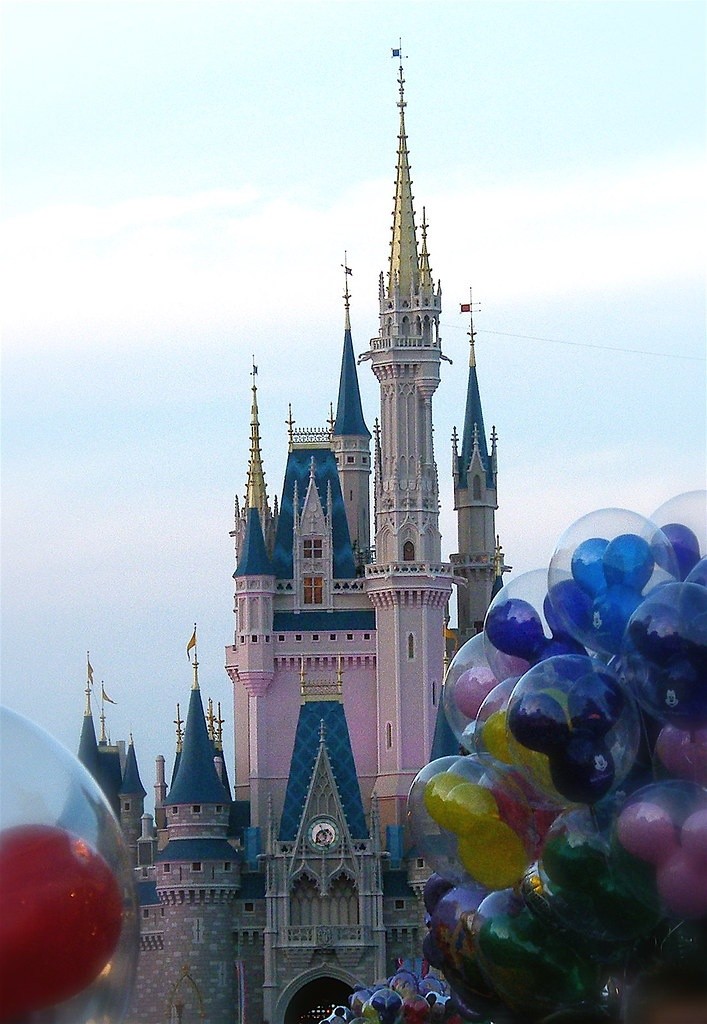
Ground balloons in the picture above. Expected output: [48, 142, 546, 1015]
[318, 968, 462, 1024]
[0, 705, 139, 1024]
[405, 488, 707, 1024]
